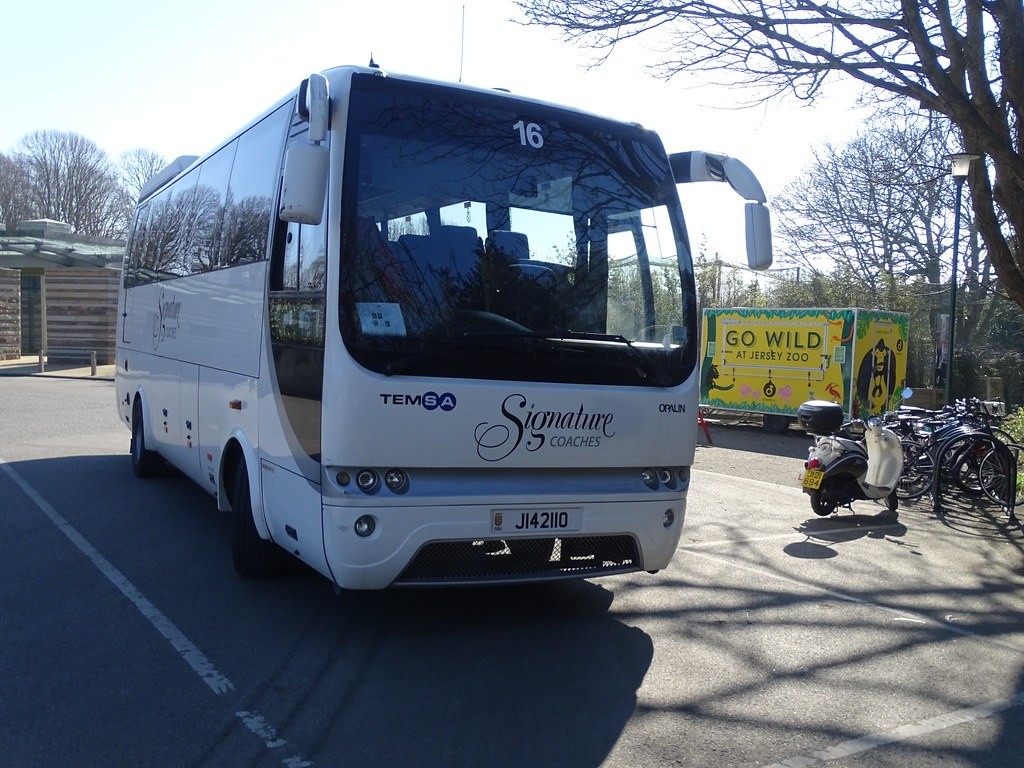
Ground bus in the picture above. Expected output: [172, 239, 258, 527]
[116, 67, 775, 595]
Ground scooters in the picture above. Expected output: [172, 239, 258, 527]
[796, 386, 914, 516]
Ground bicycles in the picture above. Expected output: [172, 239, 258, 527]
[874, 392, 1024, 522]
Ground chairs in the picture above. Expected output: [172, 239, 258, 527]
[385, 227, 573, 334]
